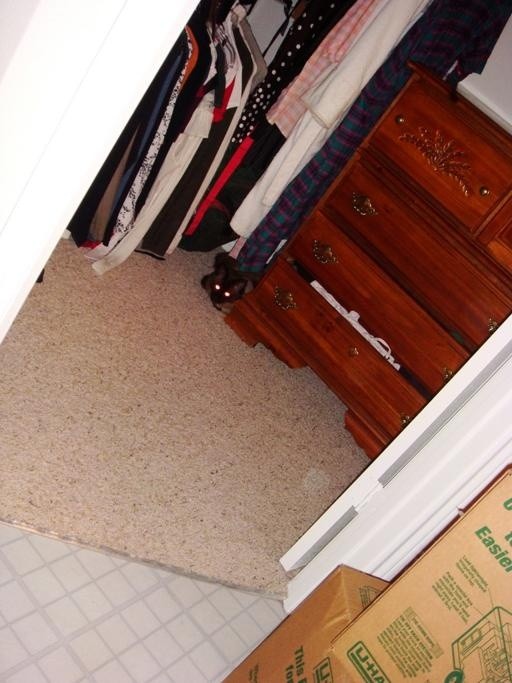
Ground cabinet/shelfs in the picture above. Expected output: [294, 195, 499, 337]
[224, 64, 512, 461]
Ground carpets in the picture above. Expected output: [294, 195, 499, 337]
[0, 239, 374, 600]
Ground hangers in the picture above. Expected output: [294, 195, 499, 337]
[186, 0, 268, 73]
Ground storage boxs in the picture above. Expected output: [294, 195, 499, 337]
[220, 564, 389, 683]
[331, 463, 512, 683]
